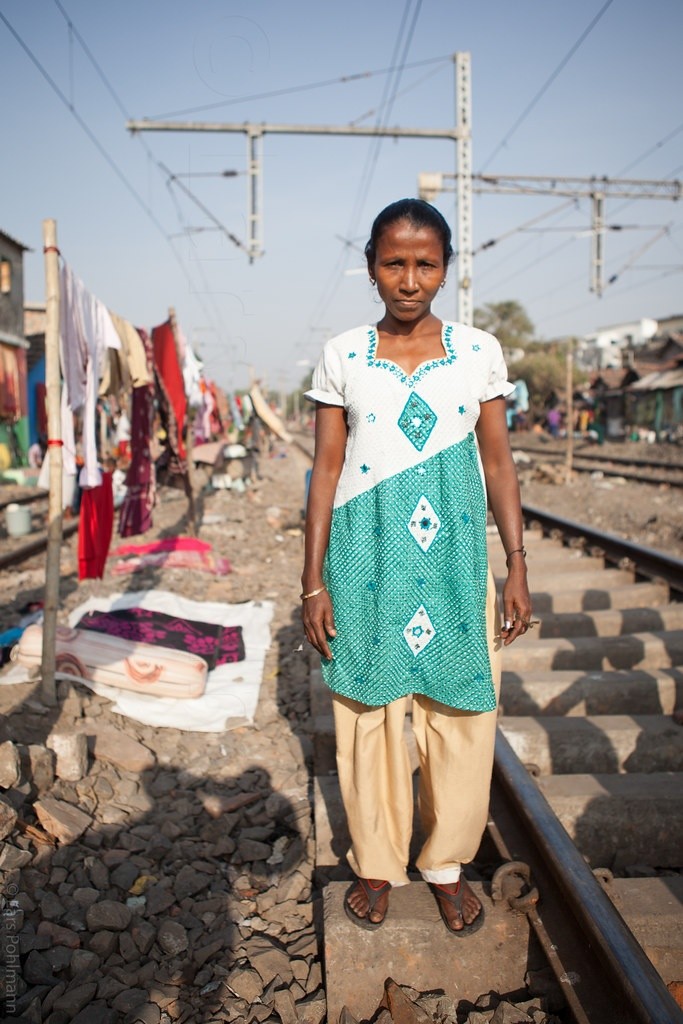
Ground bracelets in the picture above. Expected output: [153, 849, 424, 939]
[506, 549, 526, 567]
[300, 586, 326, 600]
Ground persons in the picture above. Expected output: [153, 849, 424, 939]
[545, 404, 562, 436]
[300, 197, 532, 936]
[28, 438, 44, 468]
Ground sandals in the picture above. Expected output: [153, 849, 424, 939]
[344, 878, 391, 931]
[427, 872, 485, 936]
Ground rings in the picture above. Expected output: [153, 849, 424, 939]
[515, 615, 539, 628]
